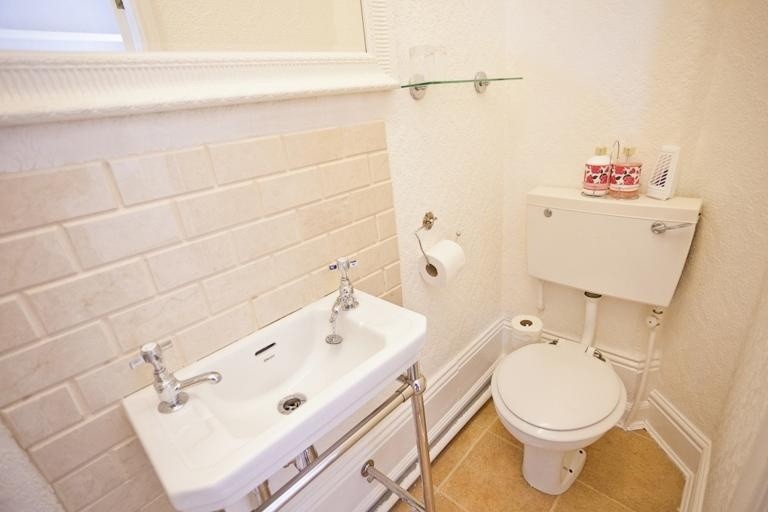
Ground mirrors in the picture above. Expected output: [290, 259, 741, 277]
[0, 0, 401, 125]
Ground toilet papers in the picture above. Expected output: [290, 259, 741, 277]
[420, 239, 464, 284]
[511, 316, 543, 348]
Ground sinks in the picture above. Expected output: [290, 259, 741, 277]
[124, 291, 427, 512]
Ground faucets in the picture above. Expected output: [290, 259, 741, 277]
[129, 340, 222, 414]
[325, 258, 358, 323]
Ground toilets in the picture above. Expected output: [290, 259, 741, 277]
[491, 185, 703, 497]
